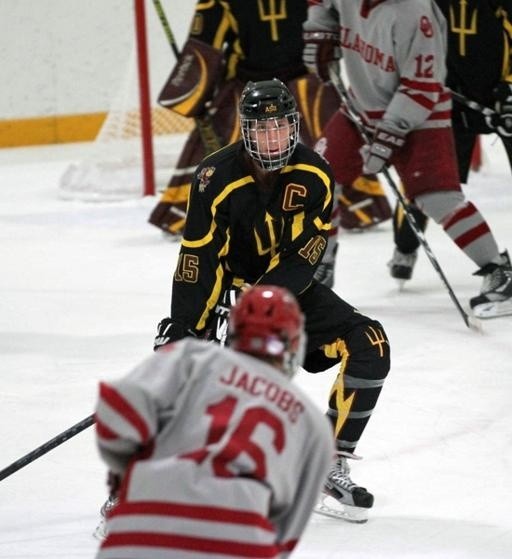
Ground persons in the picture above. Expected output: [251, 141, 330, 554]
[98, 74, 393, 509]
[148, 0, 512, 242]
[87, 285, 336, 559]
[299, 0, 512, 308]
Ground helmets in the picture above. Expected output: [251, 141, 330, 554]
[226, 284, 309, 367]
[236, 76, 302, 172]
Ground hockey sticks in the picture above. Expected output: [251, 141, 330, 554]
[327, 60, 494, 337]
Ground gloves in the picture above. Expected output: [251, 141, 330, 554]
[487, 86, 512, 134]
[301, 37, 341, 84]
[358, 121, 406, 177]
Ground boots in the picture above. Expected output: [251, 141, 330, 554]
[468, 251, 512, 307]
[324, 455, 376, 509]
[389, 246, 418, 281]
